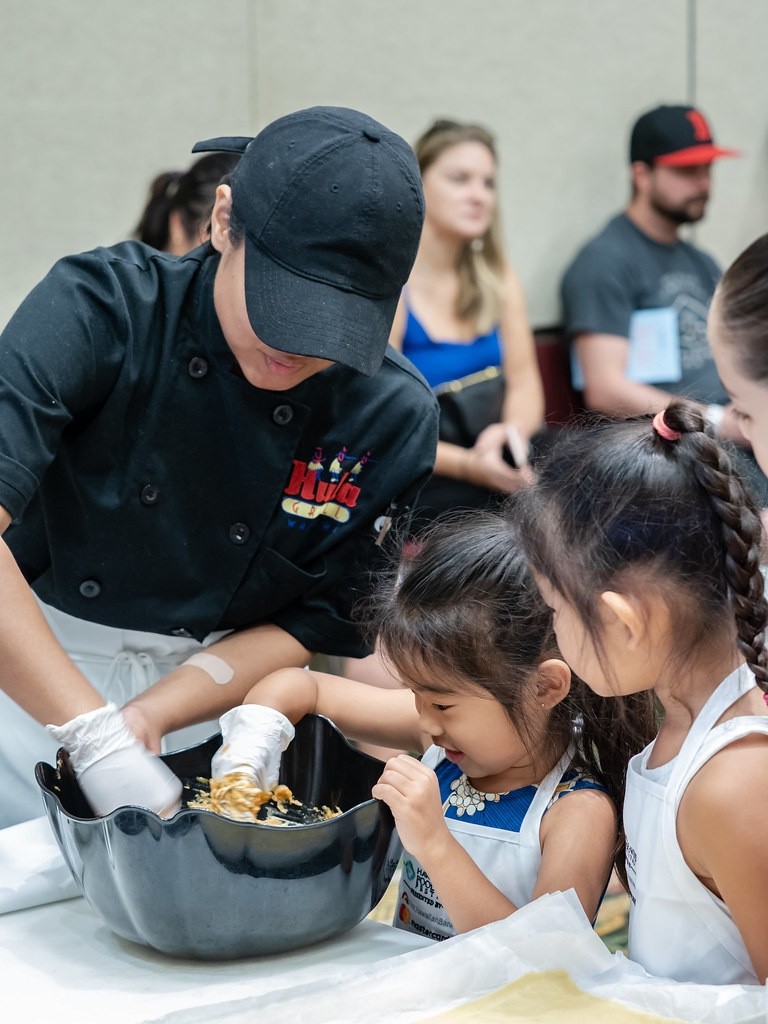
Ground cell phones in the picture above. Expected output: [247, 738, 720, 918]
[502, 425, 523, 469]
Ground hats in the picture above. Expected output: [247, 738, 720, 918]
[190, 105, 426, 378]
[630, 104, 737, 167]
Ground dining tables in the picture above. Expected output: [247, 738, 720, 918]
[0, 892, 440, 1024]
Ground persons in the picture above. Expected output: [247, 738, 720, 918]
[132, 149, 243, 257]
[502, 401, 768, 985]
[209, 509, 619, 941]
[706, 232, 768, 482]
[559, 103, 746, 416]
[1, 106, 441, 823]
[382, 117, 547, 496]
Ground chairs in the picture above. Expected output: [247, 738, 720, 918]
[529, 324, 587, 429]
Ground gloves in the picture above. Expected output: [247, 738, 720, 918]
[210, 702, 295, 793]
[44, 699, 184, 820]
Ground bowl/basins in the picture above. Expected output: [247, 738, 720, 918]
[34, 713, 404, 961]
